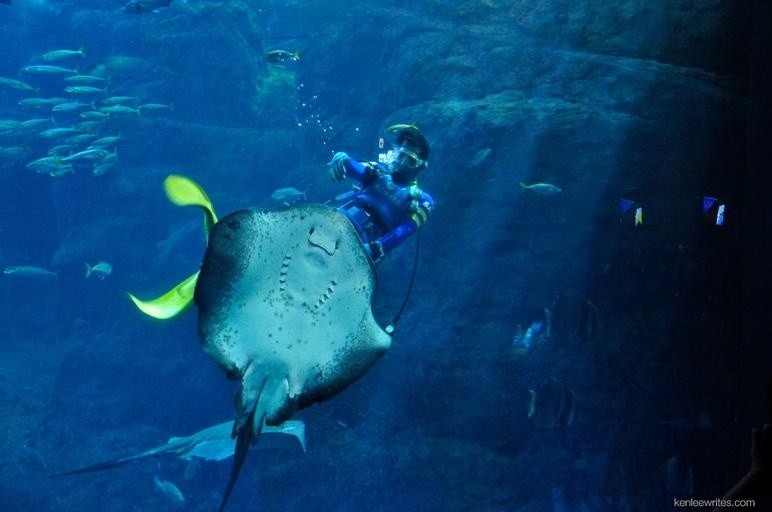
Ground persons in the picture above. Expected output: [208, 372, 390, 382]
[330, 128, 436, 263]
[712, 422, 771, 511]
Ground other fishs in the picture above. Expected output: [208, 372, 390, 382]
[1, 1, 311, 283]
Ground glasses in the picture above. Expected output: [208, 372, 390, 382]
[396, 149, 423, 168]
